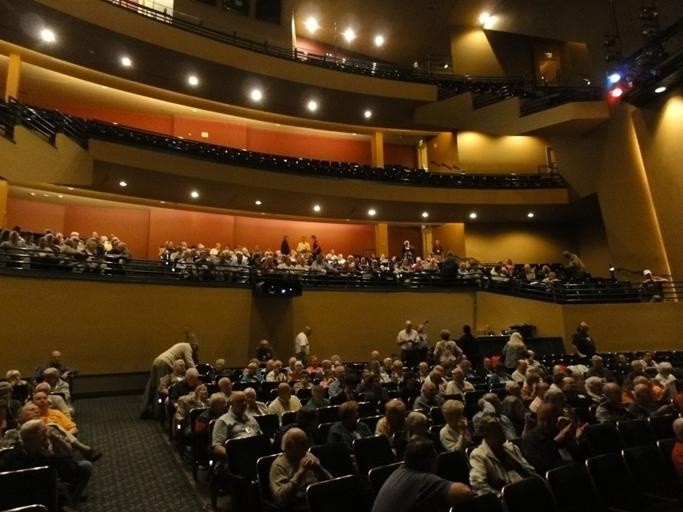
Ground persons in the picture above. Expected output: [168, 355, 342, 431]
[250, 338, 273, 361]
[310, 235, 322, 256]
[639, 268, 670, 300]
[456, 325, 481, 365]
[138, 342, 199, 422]
[501, 331, 528, 373]
[184, 328, 200, 366]
[293, 326, 311, 361]
[0, 225, 130, 277]
[280, 234, 290, 256]
[157, 352, 683, 510]
[157, 240, 571, 294]
[0, 350, 102, 511]
[417, 320, 429, 359]
[568, 320, 597, 358]
[432, 329, 464, 364]
[396, 319, 420, 362]
[294, 235, 312, 253]
[562, 250, 585, 280]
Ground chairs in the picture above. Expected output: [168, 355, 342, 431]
[1, 99, 563, 189]
[0, 375, 76, 511]
[159, 240, 633, 286]
[167, 350, 683, 511]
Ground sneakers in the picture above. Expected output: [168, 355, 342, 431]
[84, 448, 102, 460]
